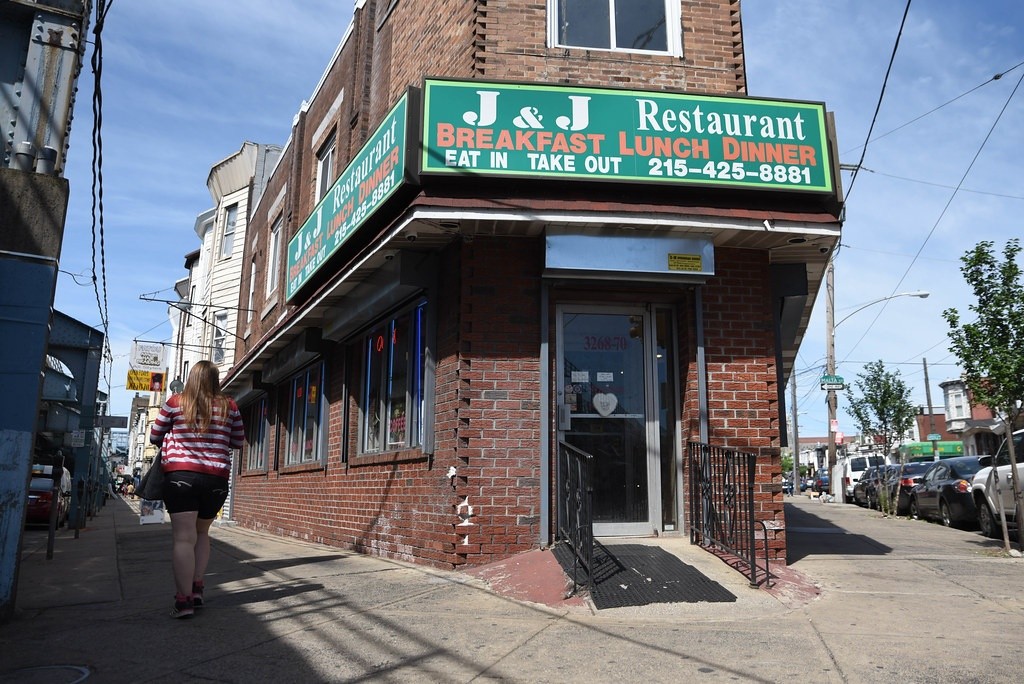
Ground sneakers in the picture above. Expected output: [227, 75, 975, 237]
[170, 590, 203, 617]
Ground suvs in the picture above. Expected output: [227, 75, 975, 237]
[811, 468, 829, 495]
[971, 427, 1024, 538]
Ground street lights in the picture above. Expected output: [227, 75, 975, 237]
[828, 290, 931, 501]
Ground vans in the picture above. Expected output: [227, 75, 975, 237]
[840, 452, 892, 505]
[23, 464, 73, 531]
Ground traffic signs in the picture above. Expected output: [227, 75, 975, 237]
[821, 383, 846, 391]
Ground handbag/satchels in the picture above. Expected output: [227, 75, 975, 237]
[133, 448, 166, 501]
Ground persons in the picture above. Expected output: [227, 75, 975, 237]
[121, 484, 125, 495]
[787, 472, 793, 497]
[124, 485, 128, 497]
[151, 375, 161, 391]
[149, 360, 245, 619]
[128, 484, 134, 495]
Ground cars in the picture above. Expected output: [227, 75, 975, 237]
[27, 476, 71, 532]
[779, 472, 814, 496]
[852, 460, 937, 517]
[909, 455, 984, 528]
